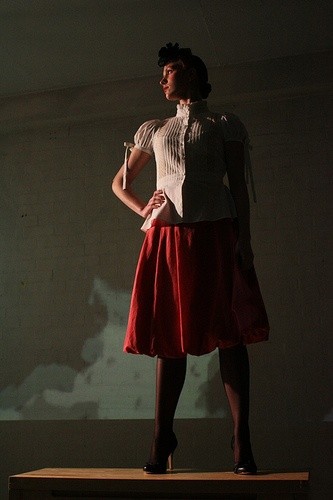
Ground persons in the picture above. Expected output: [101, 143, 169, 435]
[111, 41, 270, 475]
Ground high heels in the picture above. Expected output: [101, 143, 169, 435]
[231, 436, 257, 474]
[144, 432, 177, 474]
